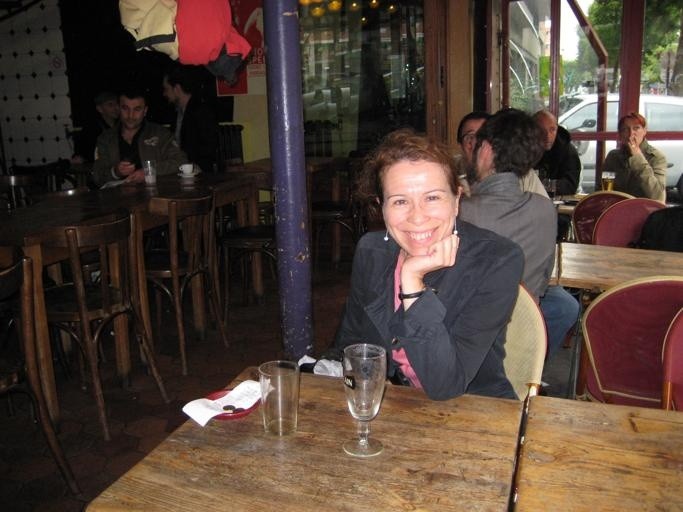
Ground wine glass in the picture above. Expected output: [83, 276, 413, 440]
[339, 342, 388, 459]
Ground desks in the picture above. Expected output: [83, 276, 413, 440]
[512, 396, 683, 512]
[86, 366, 524, 512]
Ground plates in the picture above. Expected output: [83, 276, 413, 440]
[177, 172, 197, 178]
[176, 178, 200, 182]
[200, 390, 257, 422]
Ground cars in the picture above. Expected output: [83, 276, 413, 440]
[542, 94, 593, 117]
[378, 65, 425, 100]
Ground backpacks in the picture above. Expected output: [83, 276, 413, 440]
[118, 0, 253, 88]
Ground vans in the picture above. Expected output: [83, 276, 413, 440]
[552, 93, 683, 200]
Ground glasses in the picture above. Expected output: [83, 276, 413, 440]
[459, 133, 475, 145]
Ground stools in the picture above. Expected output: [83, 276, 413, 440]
[0, 150, 365, 497]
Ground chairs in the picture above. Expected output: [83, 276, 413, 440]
[504, 189, 683, 410]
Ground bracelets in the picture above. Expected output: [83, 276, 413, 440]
[111, 166, 120, 180]
[399, 288, 426, 300]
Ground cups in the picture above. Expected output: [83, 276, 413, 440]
[600, 169, 615, 191]
[179, 164, 195, 174]
[258, 358, 300, 437]
[140, 158, 158, 185]
[181, 181, 196, 192]
[543, 177, 556, 201]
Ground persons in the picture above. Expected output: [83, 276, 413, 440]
[458, 108, 558, 307]
[79, 91, 119, 136]
[531, 109, 581, 242]
[600, 111, 667, 203]
[93, 84, 189, 184]
[452, 111, 579, 362]
[321, 126, 528, 437]
[162, 67, 224, 174]
